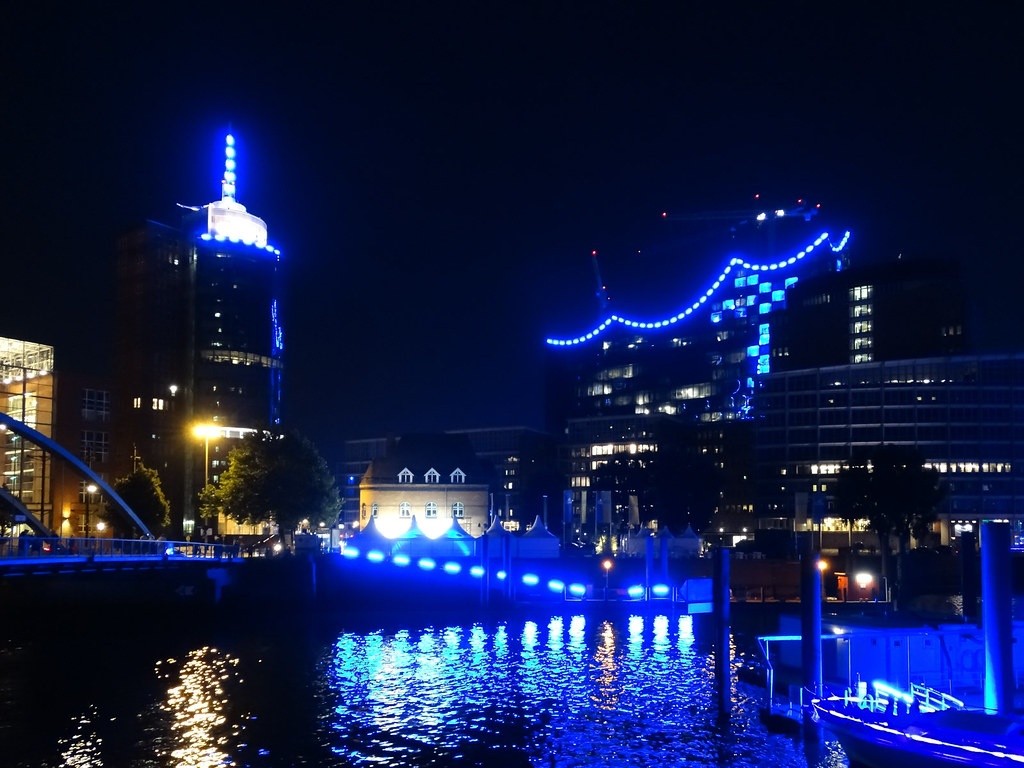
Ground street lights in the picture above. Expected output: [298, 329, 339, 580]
[859, 573, 869, 600]
[603, 558, 612, 599]
[816, 558, 830, 602]
[195, 423, 215, 495]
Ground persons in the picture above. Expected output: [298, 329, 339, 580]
[156, 533, 166, 548]
[68, 535, 75, 549]
